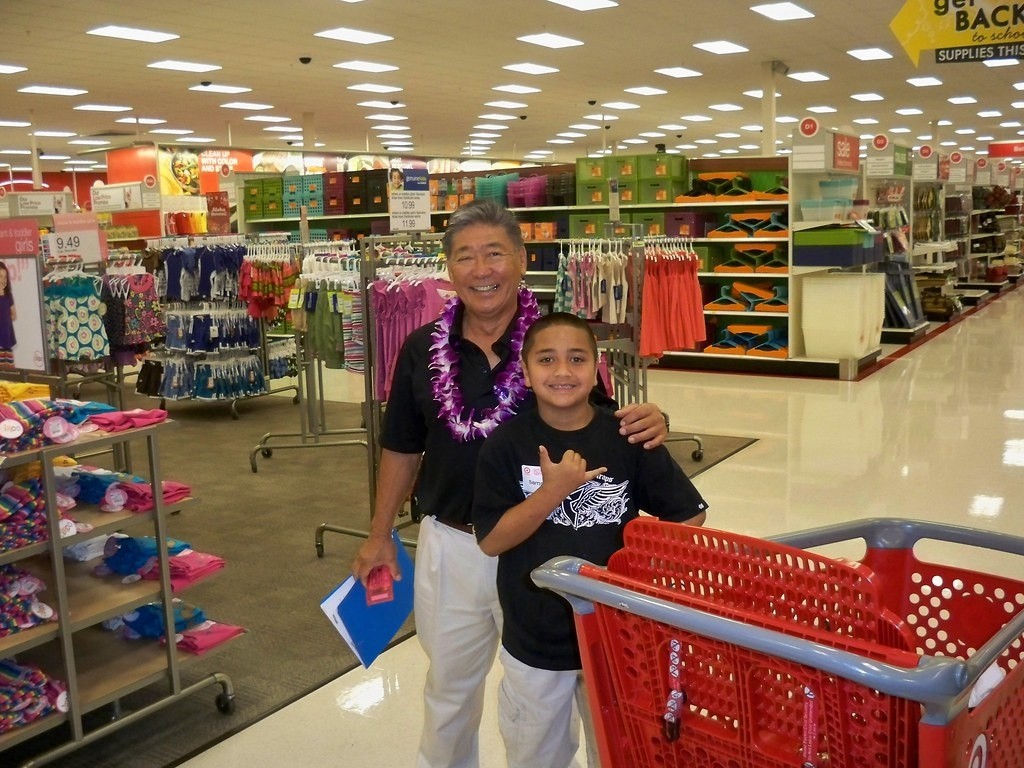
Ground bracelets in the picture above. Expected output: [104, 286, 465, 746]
[661, 410, 669, 433]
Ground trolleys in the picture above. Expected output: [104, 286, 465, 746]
[527, 517, 1024, 767]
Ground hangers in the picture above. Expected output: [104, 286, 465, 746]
[714, 244, 788, 273]
[704, 324, 789, 358]
[558, 238, 629, 263]
[683, 174, 788, 195]
[37, 232, 450, 364]
[703, 282, 788, 313]
[266, 337, 298, 359]
[643, 232, 698, 262]
[706, 211, 788, 238]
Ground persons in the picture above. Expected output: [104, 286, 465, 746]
[473, 308, 709, 768]
[348, 197, 669, 767]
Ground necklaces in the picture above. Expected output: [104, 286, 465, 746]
[428, 283, 545, 445]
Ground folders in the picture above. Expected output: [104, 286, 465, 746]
[318, 527, 415, 670]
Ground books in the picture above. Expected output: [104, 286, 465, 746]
[319, 527, 414, 670]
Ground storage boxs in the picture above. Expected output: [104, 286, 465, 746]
[243, 168, 388, 220]
[576, 156, 604, 181]
[518, 220, 559, 270]
[604, 155, 638, 181]
[792, 227, 883, 269]
[638, 153, 686, 180]
[568, 210, 703, 239]
[608, 180, 638, 205]
[638, 180, 687, 203]
[575, 181, 607, 206]
[429, 171, 575, 211]
[328, 218, 390, 251]
[800, 178, 871, 223]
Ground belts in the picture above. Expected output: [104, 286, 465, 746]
[427, 513, 476, 536]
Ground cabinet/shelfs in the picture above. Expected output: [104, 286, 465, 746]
[672, 199, 792, 362]
[2, 417, 245, 767]
[791, 169, 1023, 364]
